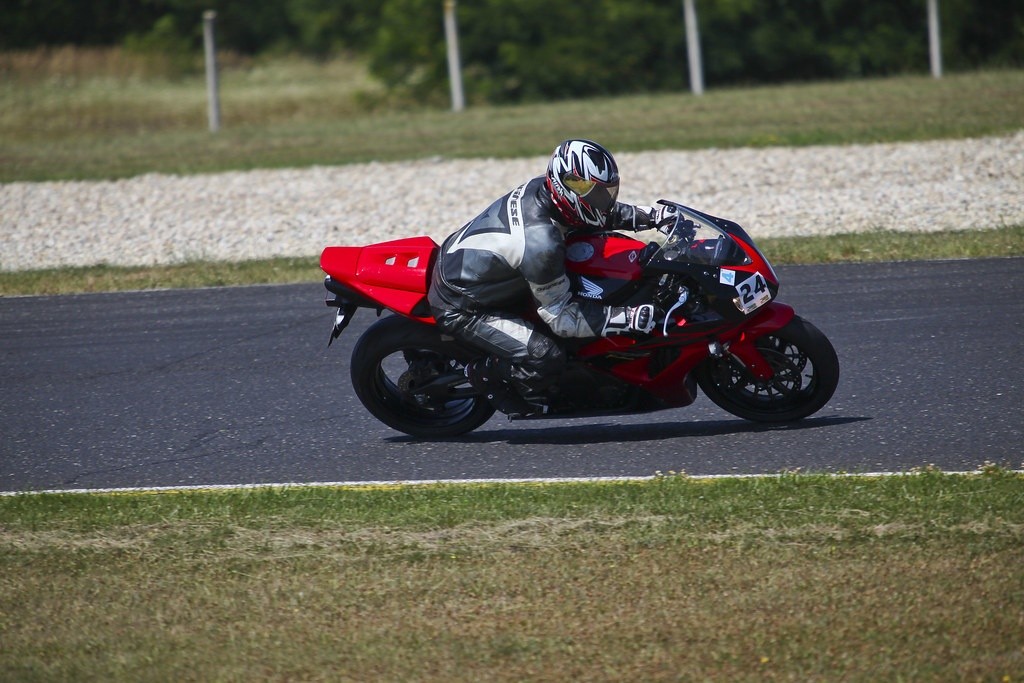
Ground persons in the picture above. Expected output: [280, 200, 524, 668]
[425, 139, 685, 416]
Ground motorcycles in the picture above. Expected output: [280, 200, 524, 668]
[318, 199, 840, 441]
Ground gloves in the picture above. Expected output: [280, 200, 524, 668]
[601, 296, 673, 343]
[633, 202, 683, 235]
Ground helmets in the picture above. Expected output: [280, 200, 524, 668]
[545, 139, 618, 233]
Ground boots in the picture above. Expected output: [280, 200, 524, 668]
[464, 355, 546, 419]
